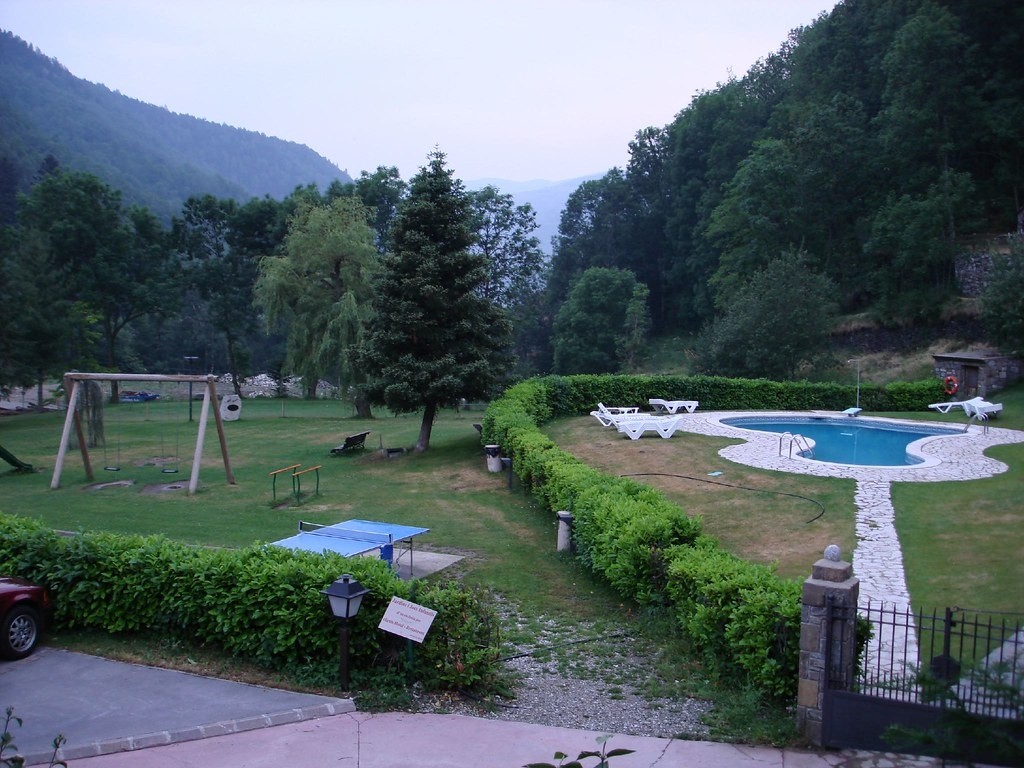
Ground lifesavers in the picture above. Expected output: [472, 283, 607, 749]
[943, 376, 958, 395]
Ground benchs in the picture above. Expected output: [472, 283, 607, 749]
[330, 431, 370, 457]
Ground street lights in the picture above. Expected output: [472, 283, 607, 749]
[184, 357, 200, 422]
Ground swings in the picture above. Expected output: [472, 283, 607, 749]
[158, 381, 180, 474]
[100, 380, 122, 472]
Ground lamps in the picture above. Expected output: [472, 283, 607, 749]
[317, 573, 370, 616]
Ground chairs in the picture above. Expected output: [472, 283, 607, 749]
[590, 411, 619, 426]
[649, 399, 698, 415]
[928, 397, 983, 413]
[598, 402, 639, 413]
[604, 413, 679, 439]
[963, 401, 1003, 422]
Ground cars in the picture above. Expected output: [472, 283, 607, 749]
[0, 575, 51, 660]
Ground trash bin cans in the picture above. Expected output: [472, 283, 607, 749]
[484, 445, 503, 473]
[555, 511, 575, 554]
